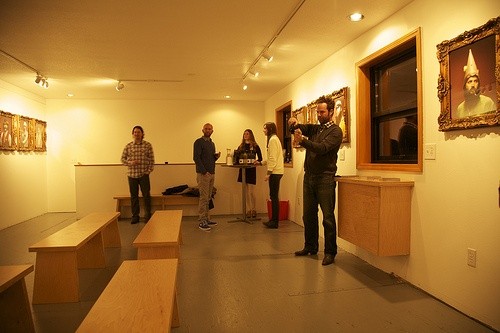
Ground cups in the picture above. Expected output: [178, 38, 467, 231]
[215, 151, 218, 156]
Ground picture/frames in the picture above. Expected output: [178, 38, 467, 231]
[0, 110, 47, 152]
[436, 16, 500, 131]
[307, 87, 351, 143]
[292, 107, 308, 148]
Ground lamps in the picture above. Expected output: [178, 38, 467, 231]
[116, 81, 124, 91]
[34, 73, 42, 83]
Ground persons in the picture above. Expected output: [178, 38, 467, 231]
[254, 122, 284, 228]
[456, 74, 496, 119]
[193, 123, 221, 231]
[235, 129, 262, 217]
[121, 125, 155, 224]
[287, 95, 343, 266]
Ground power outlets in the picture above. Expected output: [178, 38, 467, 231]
[467, 248, 476, 267]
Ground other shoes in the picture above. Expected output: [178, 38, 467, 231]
[208, 222, 217, 226]
[322, 256, 334, 265]
[199, 222, 211, 231]
[252, 210, 256, 217]
[131, 219, 139, 224]
[246, 211, 251, 218]
[263, 221, 278, 228]
[145, 218, 149, 223]
[295, 249, 317, 256]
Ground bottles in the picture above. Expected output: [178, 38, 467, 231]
[226, 149, 233, 166]
[238, 150, 256, 164]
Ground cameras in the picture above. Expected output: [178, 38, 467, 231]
[289, 126, 295, 134]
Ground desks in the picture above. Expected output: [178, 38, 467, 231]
[0, 264, 34, 333]
[221, 164, 262, 225]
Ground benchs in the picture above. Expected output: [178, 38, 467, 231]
[132, 210, 183, 259]
[75, 258, 179, 333]
[113, 195, 212, 221]
[28, 211, 121, 305]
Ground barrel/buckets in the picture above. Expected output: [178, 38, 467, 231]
[267, 199, 289, 220]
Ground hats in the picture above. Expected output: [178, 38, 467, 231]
[463, 49, 480, 82]
